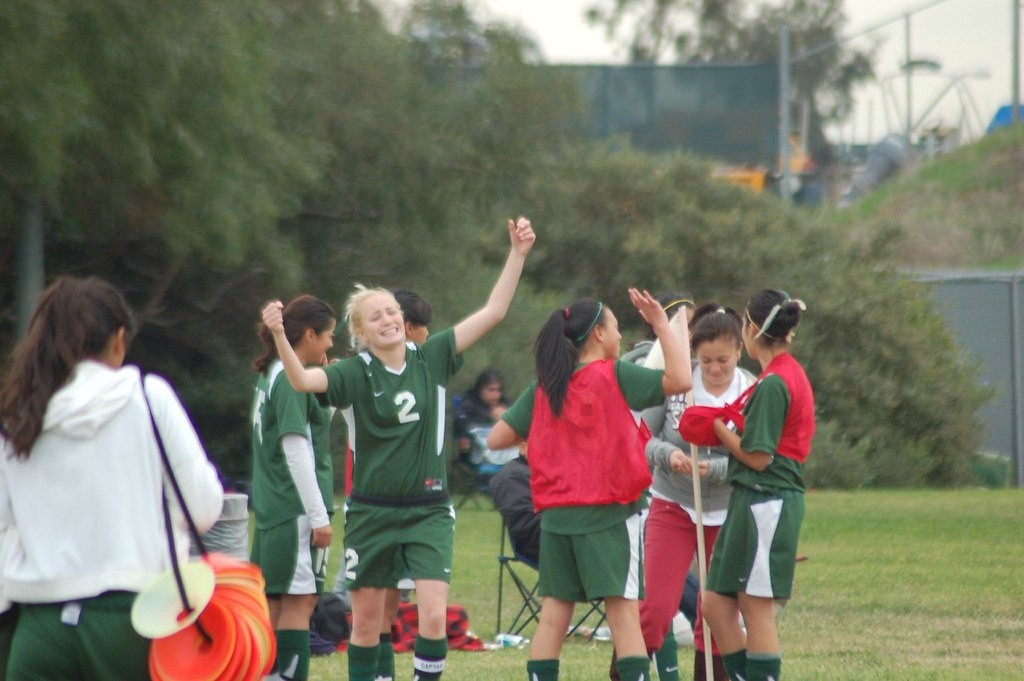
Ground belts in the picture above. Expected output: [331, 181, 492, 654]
[59, 600, 82, 625]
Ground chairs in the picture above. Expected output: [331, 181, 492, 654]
[495, 522, 614, 645]
[448, 396, 508, 513]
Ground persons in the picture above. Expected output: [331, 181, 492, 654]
[0, 275, 225, 681]
[610, 295, 757, 681]
[700, 289, 815, 681]
[489, 443, 700, 632]
[262, 219, 535, 681]
[249, 296, 337, 681]
[487, 289, 693, 681]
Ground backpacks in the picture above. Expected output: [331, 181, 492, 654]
[308, 590, 351, 640]
